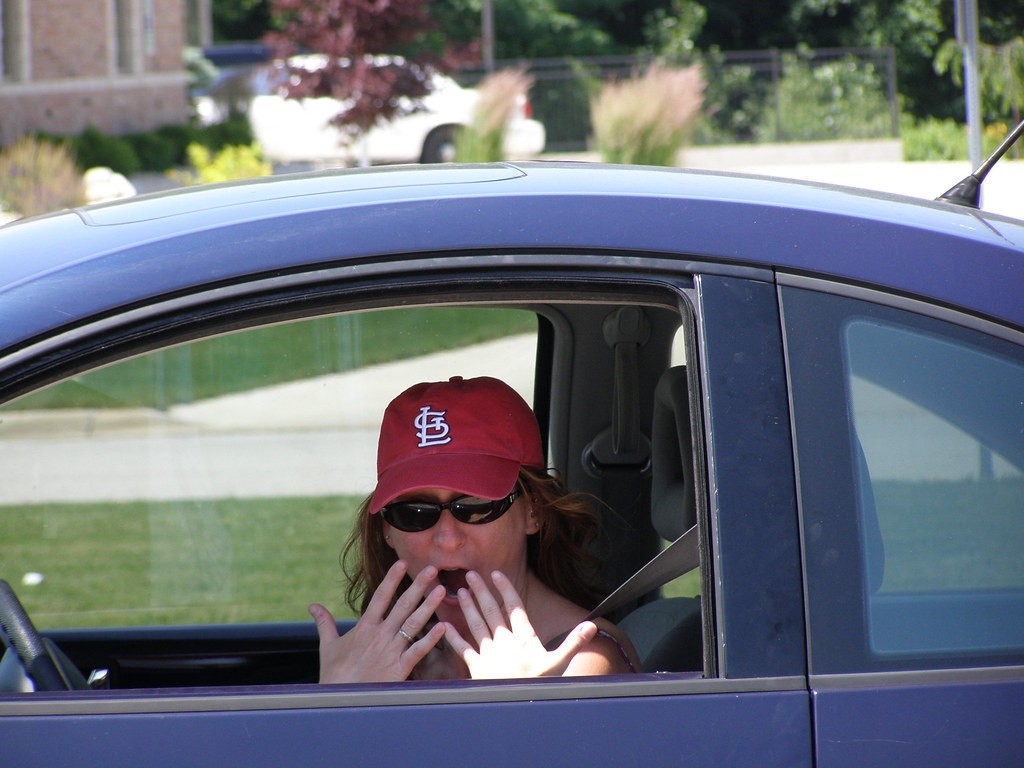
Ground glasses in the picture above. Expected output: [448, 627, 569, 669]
[381, 485, 529, 532]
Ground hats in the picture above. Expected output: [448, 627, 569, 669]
[370, 376, 547, 515]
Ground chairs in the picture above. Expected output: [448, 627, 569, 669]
[615, 363, 886, 673]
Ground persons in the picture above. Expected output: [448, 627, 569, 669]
[307, 375, 643, 684]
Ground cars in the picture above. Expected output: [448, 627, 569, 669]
[0, 162, 1024, 768]
[181, 50, 548, 171]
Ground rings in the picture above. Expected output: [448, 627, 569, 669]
[399, 629, 413, 642]
